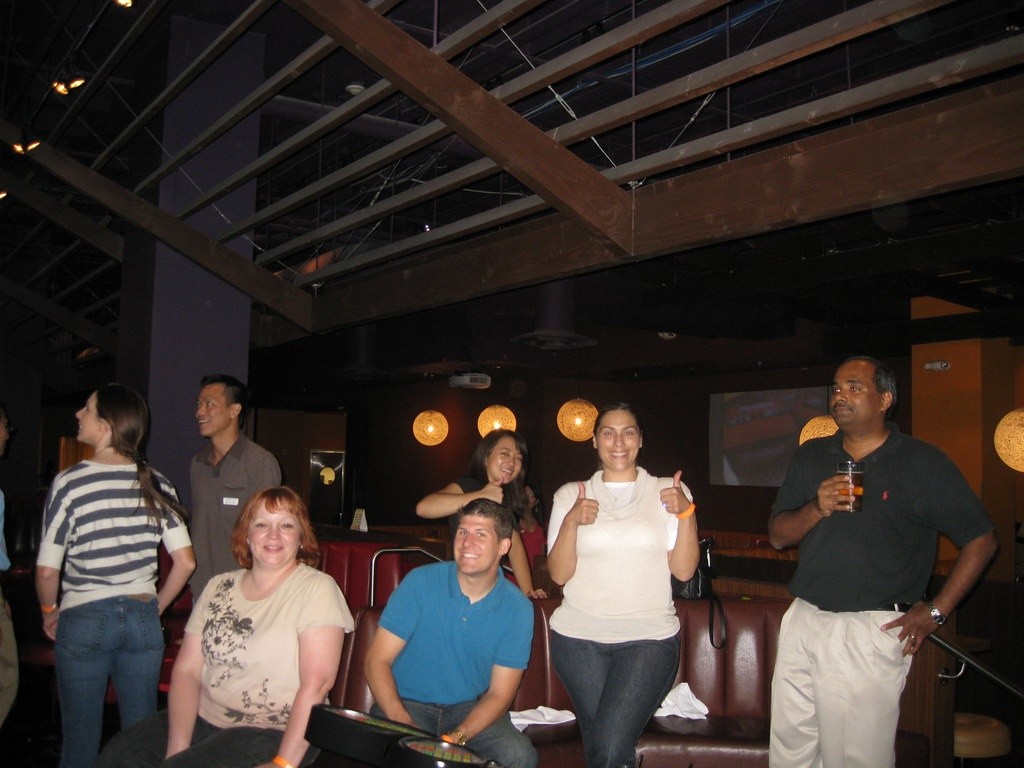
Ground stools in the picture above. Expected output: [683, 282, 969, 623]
[954, 711, 1012, 768]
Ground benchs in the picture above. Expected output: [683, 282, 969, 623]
[6, 504, 1024, 768]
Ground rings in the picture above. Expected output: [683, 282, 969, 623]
[910, 634, 917, 640]
[540, 588, 542, 592]
[663, 502, 667, 508]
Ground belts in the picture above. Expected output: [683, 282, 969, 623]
[876, 603, 911, 613]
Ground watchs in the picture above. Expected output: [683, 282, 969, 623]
[927, 599, 947, 626]
[452, 728, 468, 747]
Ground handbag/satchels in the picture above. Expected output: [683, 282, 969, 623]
[670, 536, 714, 599]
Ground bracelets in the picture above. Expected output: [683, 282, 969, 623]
[441, 735, 454, 744]
[273, 756, 295, 768]
[675, 502, 696, 520]
[41, 603, 57, 613]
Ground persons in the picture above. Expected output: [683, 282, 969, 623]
[0, 405, 18, 726]
[547, 403, 700, 768]
[416, 429, 547, 599]
[364, 498, 538, 768]
[189, 375, 280, 609]
[37, 385, 196, 768]
[769, 355, 1000, 768]
[97, 486, 355, 768]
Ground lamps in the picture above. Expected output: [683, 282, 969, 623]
[13, 122, 39, 155]
[993, 406, 1024, 473]
[412, 375, 448, 446]
[799, 369, 839, 446]
[558, 378, 599, 443]
[52, 50, 85, 96]
[477, 367, 517, 438]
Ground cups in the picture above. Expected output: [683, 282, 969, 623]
[836, 460, 863, 512]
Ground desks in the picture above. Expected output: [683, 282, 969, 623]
[1, 555, 34, 622]
[955, 630, 992, 654]
[16, 627, 62, 764]
